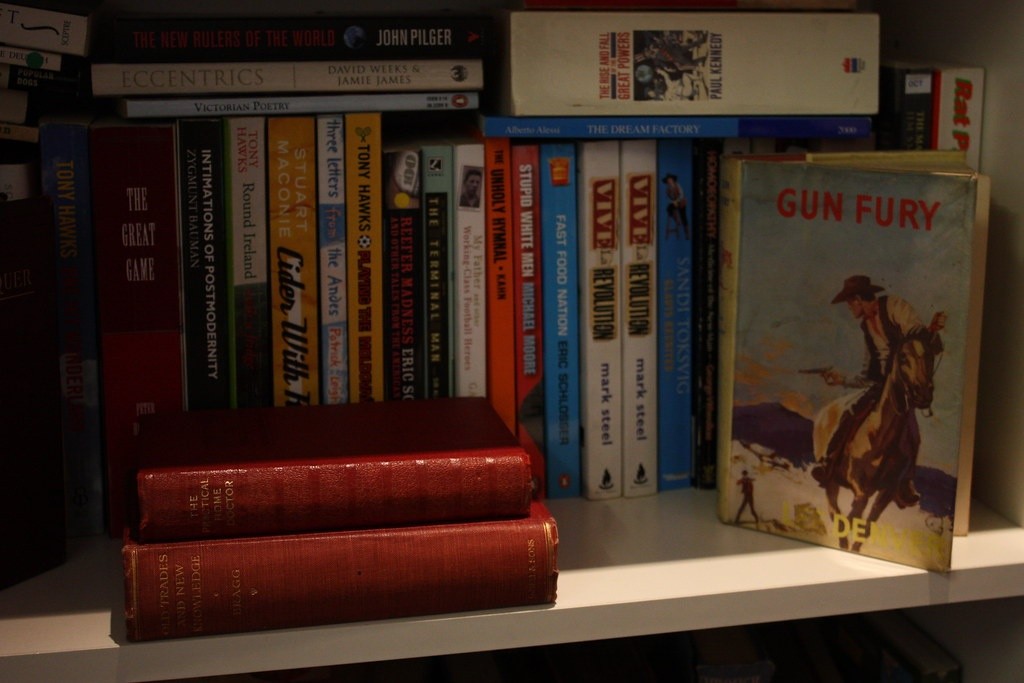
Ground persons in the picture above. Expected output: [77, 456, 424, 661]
[732, 470, 759, 524]
[810, 274, 923, 489]
[459, 170, 479, 208]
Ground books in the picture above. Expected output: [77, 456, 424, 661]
[120, 490, 559, 642]
[0, 0, 984, 542]
[715, 150, 992, 572]
[0, 195, 67, 589]
[133, 396, 533, 543]
